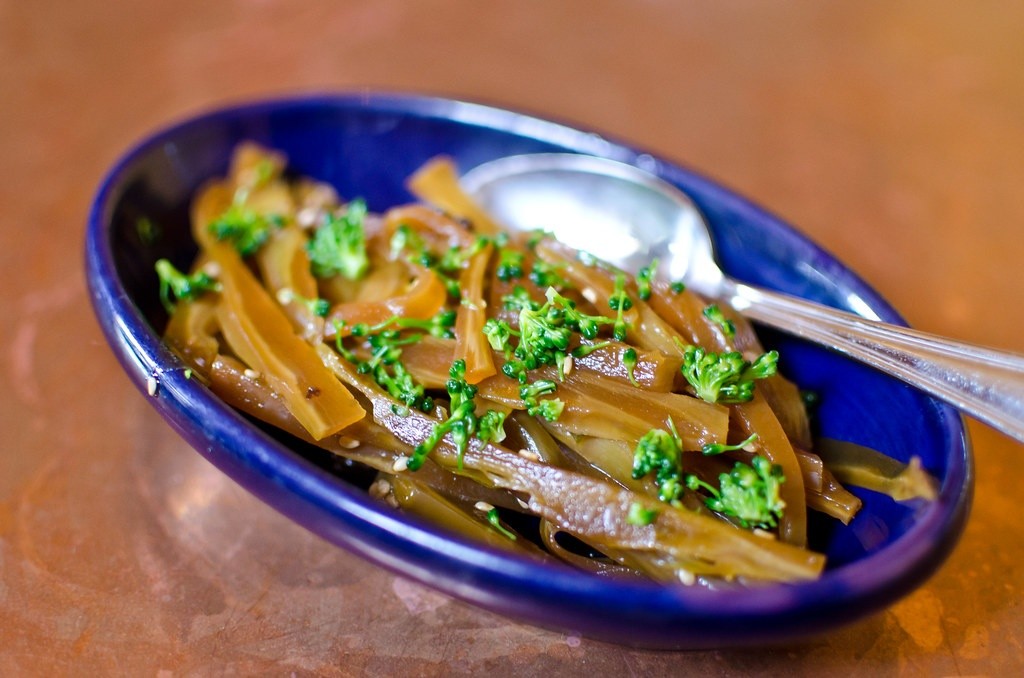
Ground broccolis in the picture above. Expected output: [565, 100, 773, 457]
[156, 165, 783, 541]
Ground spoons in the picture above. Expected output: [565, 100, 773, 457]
[455, 153, 1024, 445]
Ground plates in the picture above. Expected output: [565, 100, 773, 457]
[83, 91, 975, 654]
